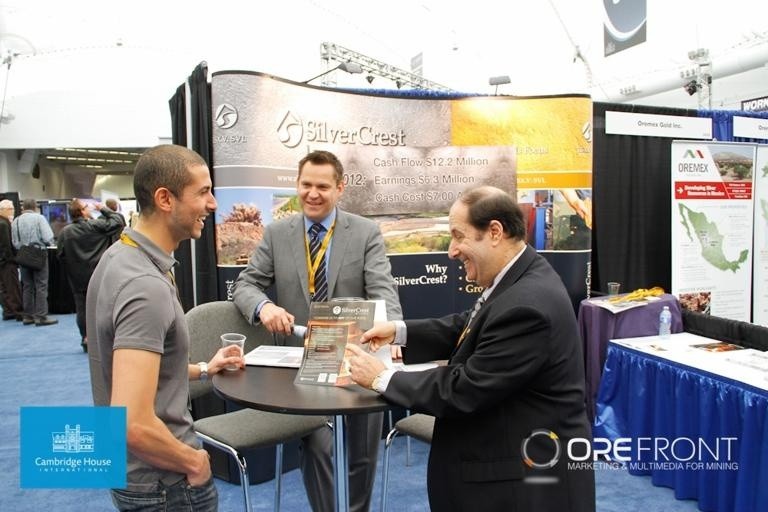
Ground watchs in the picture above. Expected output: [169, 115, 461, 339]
[370, 369, 389, 391]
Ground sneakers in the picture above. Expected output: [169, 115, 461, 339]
[4, 311, 58, 325]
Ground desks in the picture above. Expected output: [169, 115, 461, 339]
[594, 333, 768, 512]
[581, 292, 681, 398]
[213, 360, 440, 512]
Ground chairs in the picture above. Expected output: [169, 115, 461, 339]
[381, 360, 456, 512]
[185, 300, 333, 511]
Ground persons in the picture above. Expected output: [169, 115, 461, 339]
[0, 198, 126, 353]
[85, 144, 245, 512]
[232, 151, 403, 512]
[344, 186, 596, 512]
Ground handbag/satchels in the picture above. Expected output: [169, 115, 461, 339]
[14, 242, 46, 270]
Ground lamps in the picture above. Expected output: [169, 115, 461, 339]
[685, 74, 713, 110]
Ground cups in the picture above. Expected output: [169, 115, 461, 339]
[220, 333, 247, 371]
[607, 282, 621, 298]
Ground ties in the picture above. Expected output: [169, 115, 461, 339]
[308, 224, 328, 303]
[456, 298, 485, 348]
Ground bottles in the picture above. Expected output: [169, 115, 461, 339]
[659, 305, 672, 346]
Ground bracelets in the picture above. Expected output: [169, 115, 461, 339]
[198, 361, 209, 383]
